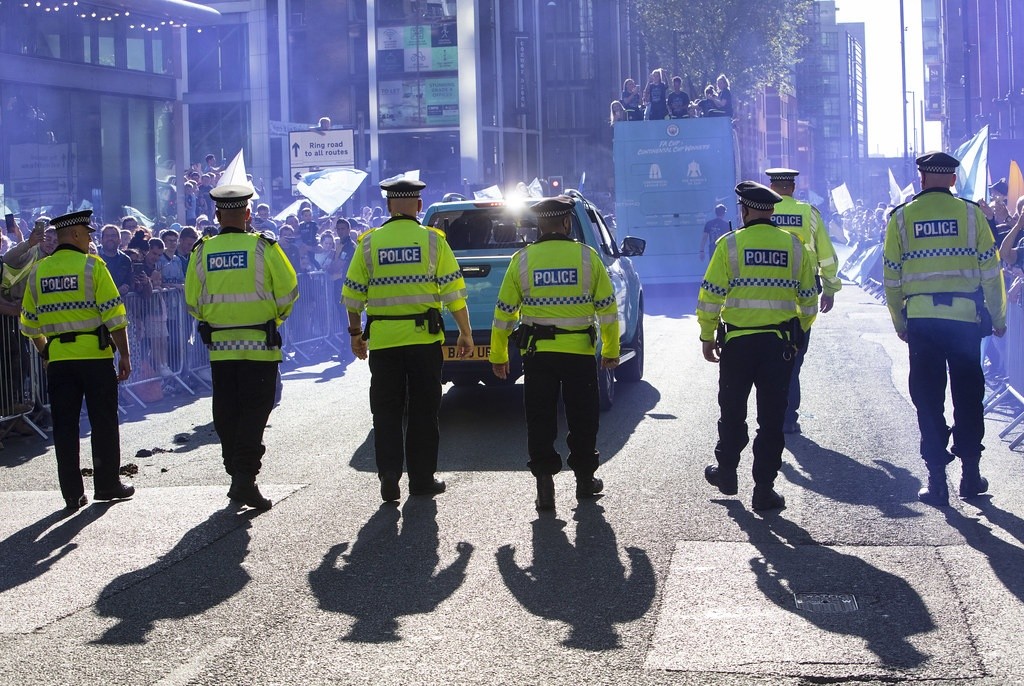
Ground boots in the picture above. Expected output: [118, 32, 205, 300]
[956, 454, 989, 498]
[227, 471, 272, 508]
[704, 464, 739, 495]
[917, 471, 949, 506]
[574, 471, 604, 498]
[534, 476, 556, 510]
[751, 481, 786, 511]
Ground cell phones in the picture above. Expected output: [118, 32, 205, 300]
[285, 237, 296, 244]
[5, 214, 15, 233]
[133, 262, 144, 276]
[35, 221, 44, 236]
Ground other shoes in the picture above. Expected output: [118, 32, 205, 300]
[408, 478, 447, 496]
[93, 481, 136, 501]
[783, 422, 801, 435]
[380, 482, 400, 501]
[65, 493, 88, 510]
[0, 378, 182, 435]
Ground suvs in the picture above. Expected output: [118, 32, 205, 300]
[419, 187, 647, 412]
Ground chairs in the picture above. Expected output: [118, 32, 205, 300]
[492, 225, 517, 243]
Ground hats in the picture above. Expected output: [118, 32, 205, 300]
[765, 168, 800, 181]
[734, 181, 783, 210]
[914, 150, 960, 175]
[380, 177, 427, 198]
[209, 183, 254, 209]
[528, 194, 578, 219]
[48, 209, 97, 234]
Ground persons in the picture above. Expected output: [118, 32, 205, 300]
[699, 203, 732, 261]
[338, 180, 476, 502]
[184, 184, 299, 511]
[828, 192, 914, 247]
[89, 215, 200, 408]
[883, 152, 1009, 506]
[19, 209, 134, 509]
[604, 68, 737, 122]
[309, 117, 331, 136]
[184, 147, 280, 241]
[695, 181, 818, 513]
[488, 195, 621, 510]
[278, 198, 391, 362]
[0, 204, 60, 436]
[978, 177, 1024, 383]
[766, 167, 842, 433]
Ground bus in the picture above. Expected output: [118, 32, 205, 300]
[611, 113, 744, 296]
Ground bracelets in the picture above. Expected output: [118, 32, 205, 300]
[348, 327, 362, 336]
